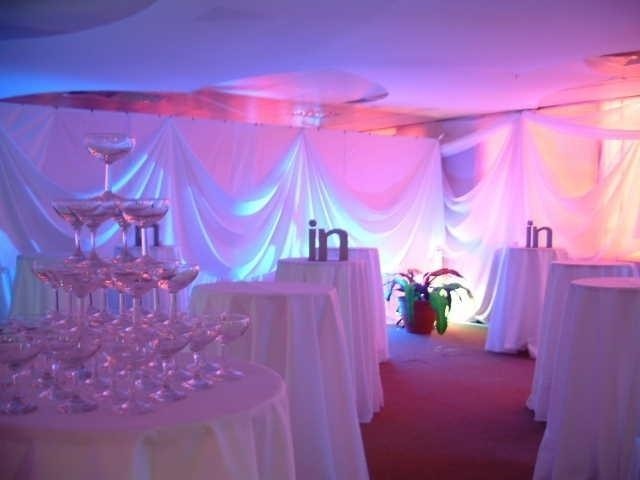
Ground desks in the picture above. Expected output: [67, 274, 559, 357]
[192, 281, 370, 480]
[2, 354, 295, 479]
[483, 246, 570, 353]
[308, 248, 389, 363]
[274, 256, 384, 421]
[532, 278, 640, 480]
[527, 261, 636, 422]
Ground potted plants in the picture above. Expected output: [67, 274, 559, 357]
[383, 267, 471, 336]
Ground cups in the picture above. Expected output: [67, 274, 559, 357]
[53, 265, 79, 330]
[145, 282, 168, 321]
[68, 200, 120, 268]
[59, 268, 106, 336]
[183, 313, 225, 375]
[30, 258, 61, 319]
[52, 201, 87, 264]
[84, 132, 136, 202]
[158, 262, 201, 330]
[204, 312, 252, 382]
[109, 268, 157, 332]
[111, 211, 138, 264]
[119, 197, 170, 266]
[88, 283, 116, 323]
[1, 311, 183, 416]
[104, 284, 132, 328]
